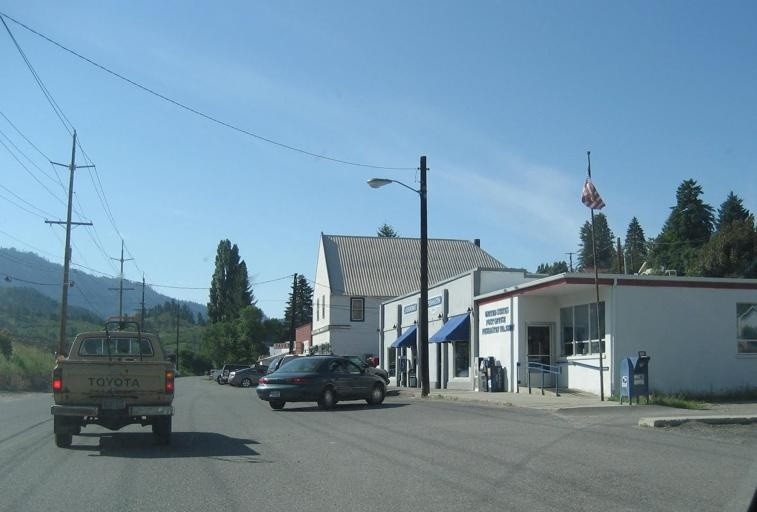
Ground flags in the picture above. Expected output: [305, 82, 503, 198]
[581, 159, 606, 211]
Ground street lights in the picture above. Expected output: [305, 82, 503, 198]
[367, 153, 429, 398]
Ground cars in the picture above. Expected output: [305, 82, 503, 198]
[210, 354, 389, 411]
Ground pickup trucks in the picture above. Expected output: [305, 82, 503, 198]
[51, 326, 176, 452]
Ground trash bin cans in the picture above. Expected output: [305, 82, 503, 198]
[620, 356, 650, 405]
